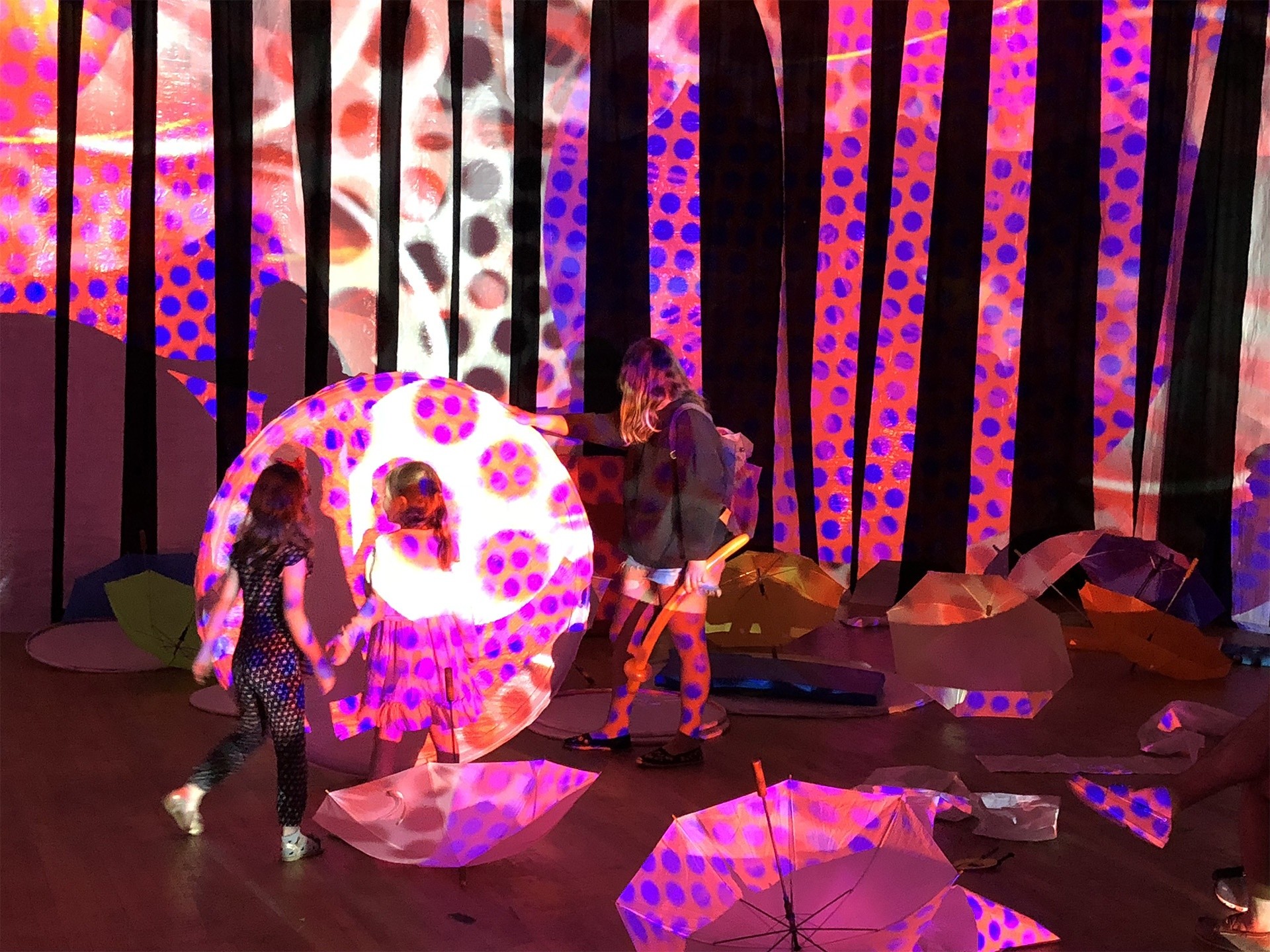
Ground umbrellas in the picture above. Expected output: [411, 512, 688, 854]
[24, 426, 1230, 951]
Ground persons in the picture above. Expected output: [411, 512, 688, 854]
[325, 462, 482, 785]
[162, 462, 336, 863]
[1064, 697, 1270, 952]
[496, 337, 734, 751]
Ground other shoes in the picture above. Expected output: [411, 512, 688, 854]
[636, 747, 702, 767]
[1215, 877, 1248, 912]
[563, 732, 631, 750]
[1065, 774, 1172, 849]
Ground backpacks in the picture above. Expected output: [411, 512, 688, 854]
[668, 404, 763, 539]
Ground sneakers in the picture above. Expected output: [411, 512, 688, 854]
[163, 782, 206, 835]
[1194, 912, 1270, 952]
[281, 825, 320, 861]
